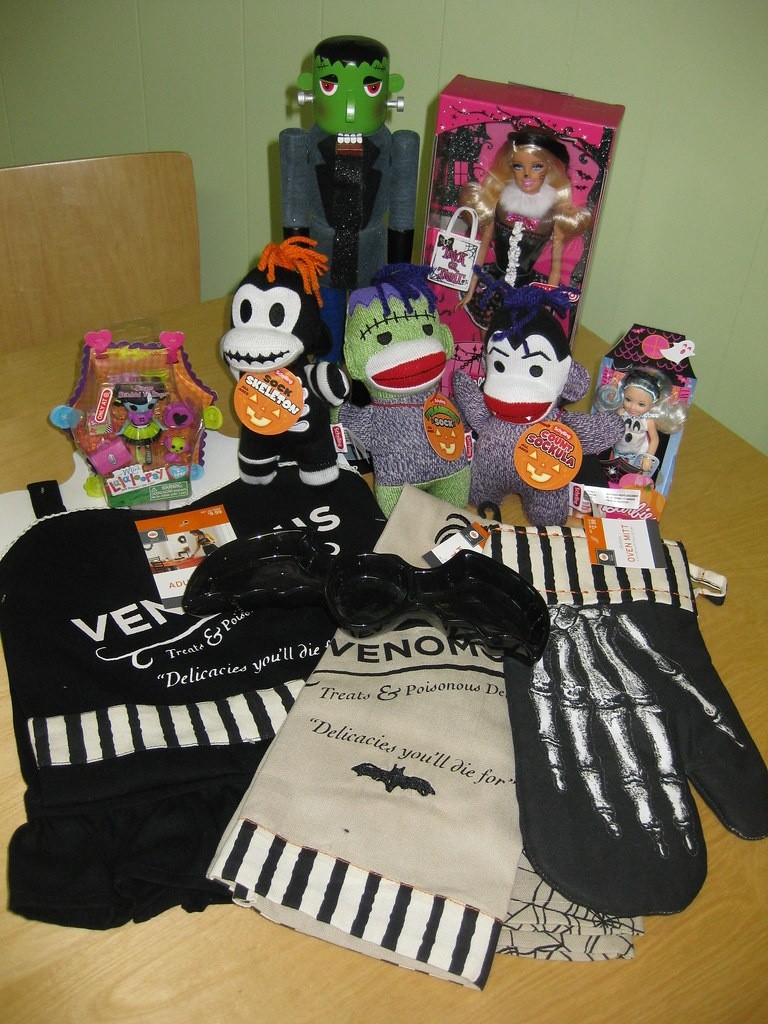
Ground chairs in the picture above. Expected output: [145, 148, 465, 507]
[0, 152, 202, 357]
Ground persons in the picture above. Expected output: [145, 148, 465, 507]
[189, 529, 218, 559]
[611, 370, 672, 471]
[454, 125, 591, 344]
[665, 471, 668, 471]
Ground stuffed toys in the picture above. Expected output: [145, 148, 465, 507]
[218, 236, 628, 528]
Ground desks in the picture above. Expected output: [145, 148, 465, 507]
[0, 294, 768, 1024]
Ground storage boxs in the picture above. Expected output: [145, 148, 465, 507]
[420, 74, 626, 397]
[590, 324, 696, 497]
